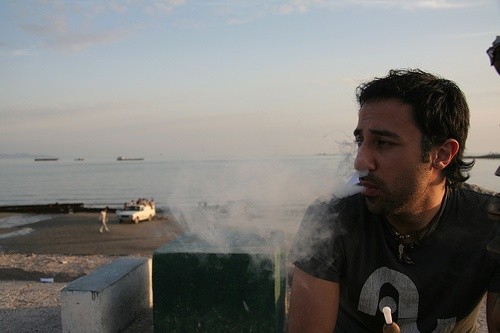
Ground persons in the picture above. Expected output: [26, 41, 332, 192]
[98, 206, 111, 233]
[124, 198, 155, 210]
[288, 67, 500, 333]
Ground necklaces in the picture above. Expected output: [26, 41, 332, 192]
[383, 182, 449, 264]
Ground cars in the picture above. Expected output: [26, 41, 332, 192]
[119, 205, 156, 224]
